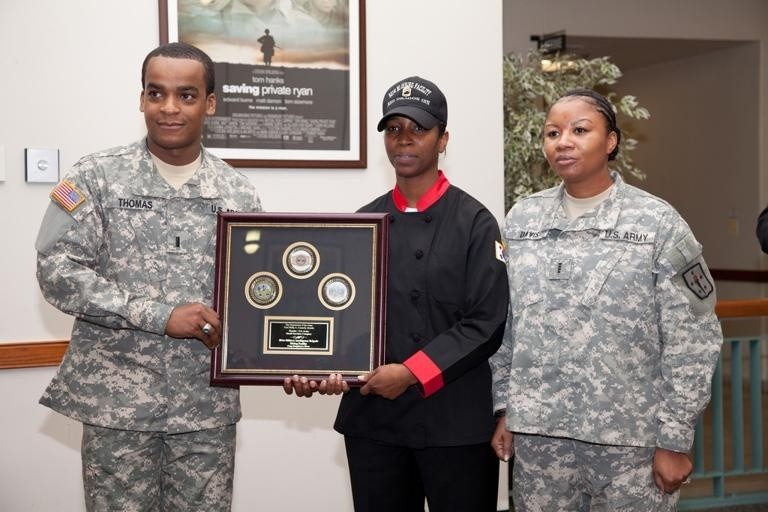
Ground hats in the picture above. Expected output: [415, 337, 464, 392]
[377, 77, 447, 131]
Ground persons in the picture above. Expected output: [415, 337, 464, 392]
[486, 88, 724, 512]
[279, 73, 510, 512]
[33, 40, 266, 512]
[259, 27, 276, 66]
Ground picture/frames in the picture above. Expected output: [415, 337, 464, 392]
[156, 0, 368, 169]
[208, 210, 391, 389]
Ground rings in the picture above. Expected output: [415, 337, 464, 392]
[681, 478, 690, 485]
[201, 323, 212, 334]
